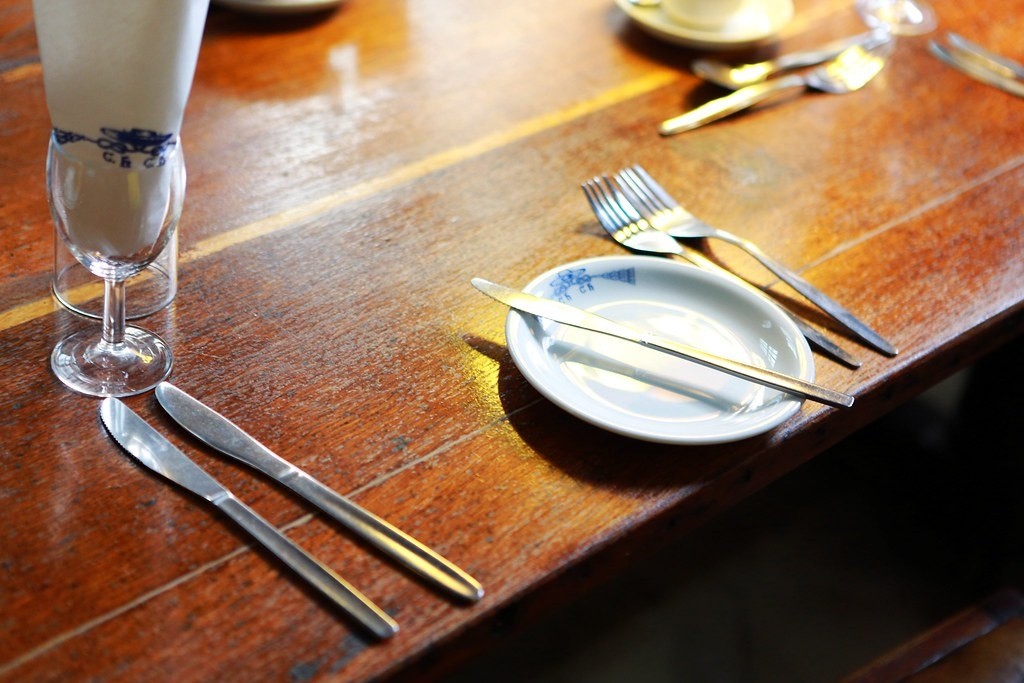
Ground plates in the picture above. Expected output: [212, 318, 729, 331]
[615, 1, 794, 47]
[506, 255, 816, 444]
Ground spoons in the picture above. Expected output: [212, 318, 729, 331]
[855, 2, 937, 36]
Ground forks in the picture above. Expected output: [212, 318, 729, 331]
[692, 26, 893, 91]
[613, 164, 900, 356]
[658, 46, 884, 136]
[582, 173, 862, 370]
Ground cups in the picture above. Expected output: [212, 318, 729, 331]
[659, 0, 746, 28]
[53, 218, 177, 319]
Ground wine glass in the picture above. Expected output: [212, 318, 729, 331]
[45, 130, 187, 399]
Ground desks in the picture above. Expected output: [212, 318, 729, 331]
[0, 0, 1024, 683]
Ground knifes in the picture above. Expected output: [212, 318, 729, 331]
[948, 34, 1024, 82]
[99, 396, 400, 639]
[471, 277, 855, 411]
[929, 40, 1024, 98]
[155, 381, 484, 600]
